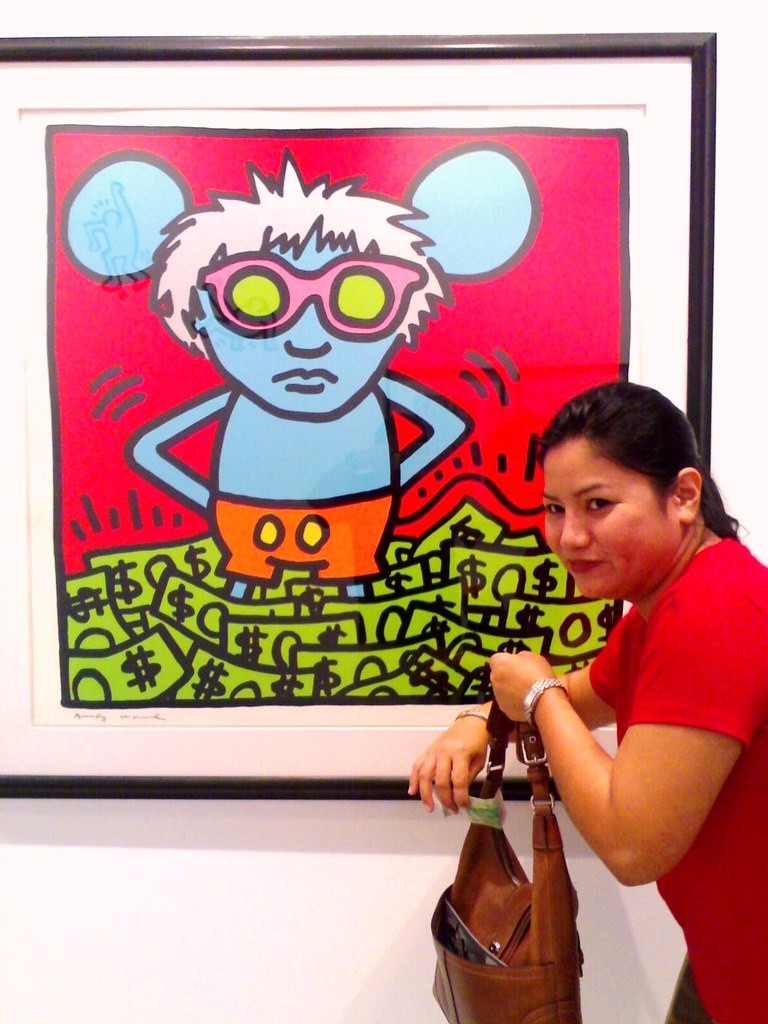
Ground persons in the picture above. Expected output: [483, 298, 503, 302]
[407, 381, 768, 1024]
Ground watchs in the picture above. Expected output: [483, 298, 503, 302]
[524, 679, 569, 727]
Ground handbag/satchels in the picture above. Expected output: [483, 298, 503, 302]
[430, 701, 584, 1024]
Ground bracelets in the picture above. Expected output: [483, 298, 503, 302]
[456, 712, 488, 720]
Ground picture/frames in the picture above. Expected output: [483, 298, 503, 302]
[0, 30, 718, 799]
